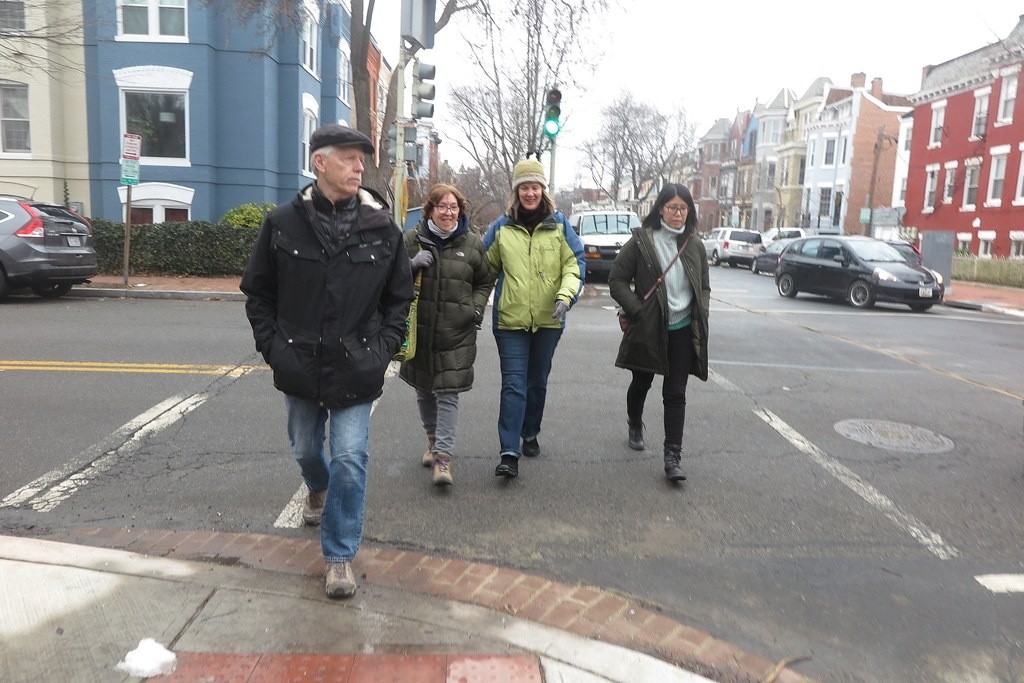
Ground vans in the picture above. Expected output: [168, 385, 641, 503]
[761, 227, 817, 247]
[569, 212, 643, 277]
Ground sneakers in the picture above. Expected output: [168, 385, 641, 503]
[432, 452, 454, 485]
[302, 485, 326, 524]
[494, 453, 518, 477]
[423, 434, 437, 466]
[522, 437, 540, 455]
[324, 557, 357, 598]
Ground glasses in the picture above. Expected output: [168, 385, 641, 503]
[433, 202, 460, 214]
[662, 203, 690, 215]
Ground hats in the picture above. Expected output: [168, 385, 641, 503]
[310, 129, 376, 154]
[511, 159, 546, 191]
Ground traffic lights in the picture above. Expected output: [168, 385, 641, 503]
[401, 0, 435, 50]
[543, 88, 562, 138]
[411, 59, 436, 120]
[389, 126, 416, 163]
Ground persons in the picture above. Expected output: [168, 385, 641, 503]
[240, 125, 416, 599]
[608, 183, 711, 481]
[483, 160, 586, 476]
[398, 184, 495, 485]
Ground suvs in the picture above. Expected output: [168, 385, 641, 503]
[0, 198, 101, 300]
[700, 226, 765, 267]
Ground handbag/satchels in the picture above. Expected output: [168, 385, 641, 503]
[391, 297, 418, 361]
[617, 302, 630, 331]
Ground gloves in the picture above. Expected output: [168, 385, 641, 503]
[410, 249, 434, 271]
[551, 300, 569, 323]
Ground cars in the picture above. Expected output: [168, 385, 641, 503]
[750, 238, 796, 274]
[776, 236, 945, 312]
[890, 242, 923, 265]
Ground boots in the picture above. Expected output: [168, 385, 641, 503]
[663, 442, 687, 481]
[626, 416, 645, 450]
